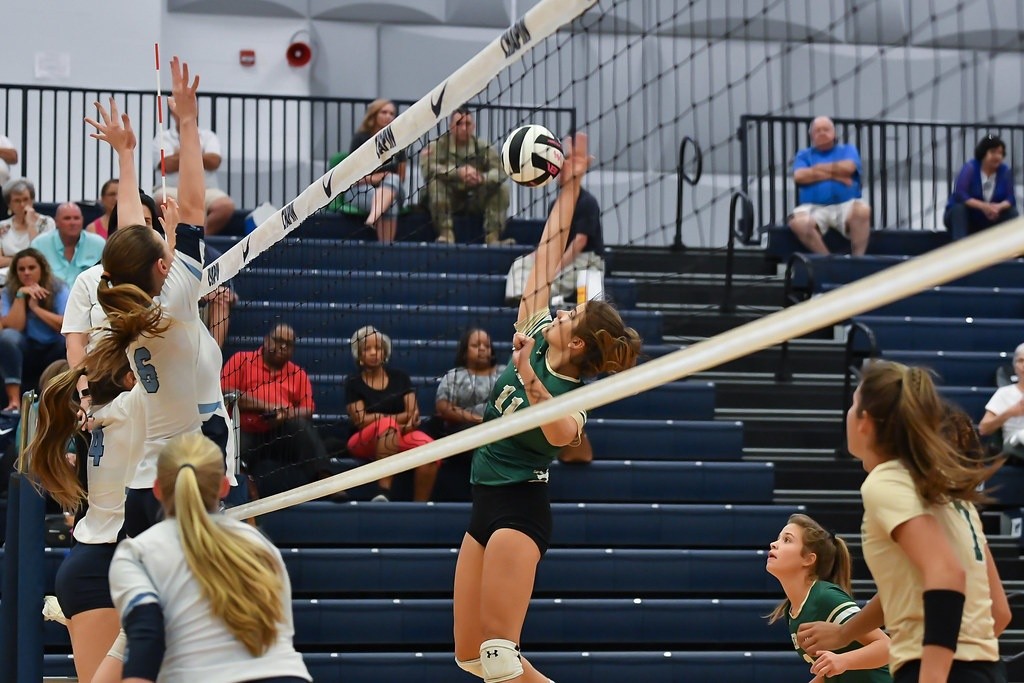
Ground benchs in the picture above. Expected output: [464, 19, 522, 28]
[0, 235, 1024, 683]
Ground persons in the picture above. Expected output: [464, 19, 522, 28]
[222, 323, 349, 499]
[85, 55, 229, 683]
[150, 107, 232, 235]
[943, 133, 1019, 240]
[0, 133, 238, 522]
[455, 131, 639, 682]
[107, 429, 315, 683]
[337, 327, 442, 503]
[979, 342, 1024, 535]
[435, 327, 510, 502]
[760, 513, 893, 683]
[795, 360, 1010, 683]
[13, 333, 145, 682]
[345, 96, 408, 242]
[546, 164, 604, 300]
[420, 108, 518, 244]
[789, 113, 871, 256]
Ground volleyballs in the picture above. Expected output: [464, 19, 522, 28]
[501, 124, 564, 188]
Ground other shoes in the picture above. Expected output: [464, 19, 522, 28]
[329, 491, 349, 502]
[484, 232, 516, 245]
[434, 228, 456, 243]
[372, 487, 395, 501]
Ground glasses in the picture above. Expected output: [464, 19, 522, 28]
[271, 335, 293, 347]
